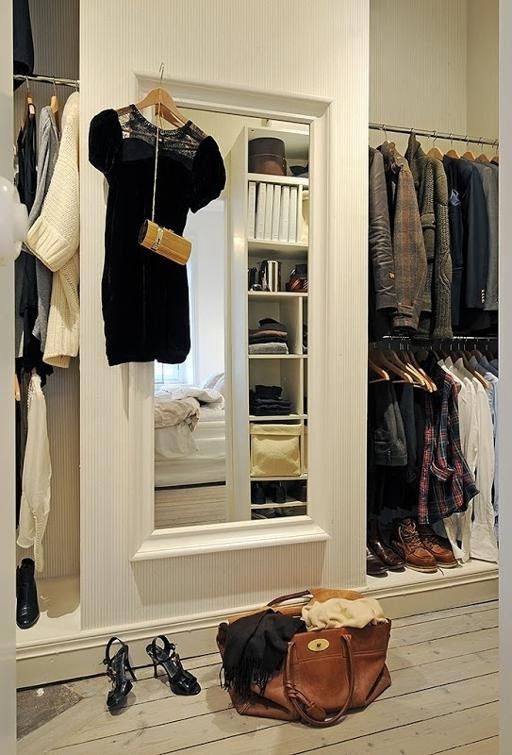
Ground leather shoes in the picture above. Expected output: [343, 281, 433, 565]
[366, 518, 406, 570]
[367, 544, 388, 576]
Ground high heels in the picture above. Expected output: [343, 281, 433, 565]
[145, 631, 203, 696]
[101, 636, 137, 709]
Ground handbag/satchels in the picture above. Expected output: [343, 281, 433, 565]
[138, 101, 193, 267]
[216, 586, 393, 730]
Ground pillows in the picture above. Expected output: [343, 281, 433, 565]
[199, 369, 222, 389]
[212, 376, 226, 398]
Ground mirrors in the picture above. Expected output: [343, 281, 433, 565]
[132, 75, 333, 542]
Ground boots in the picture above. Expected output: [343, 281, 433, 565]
[15, 558, 41, 629]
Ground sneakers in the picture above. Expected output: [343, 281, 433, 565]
[417, 524, 458, 570]
[390, 517, 439, 573]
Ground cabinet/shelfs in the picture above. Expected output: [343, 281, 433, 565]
[222, 125, 310, 520]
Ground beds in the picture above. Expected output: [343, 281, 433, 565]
[154, 370, 226, 490]
